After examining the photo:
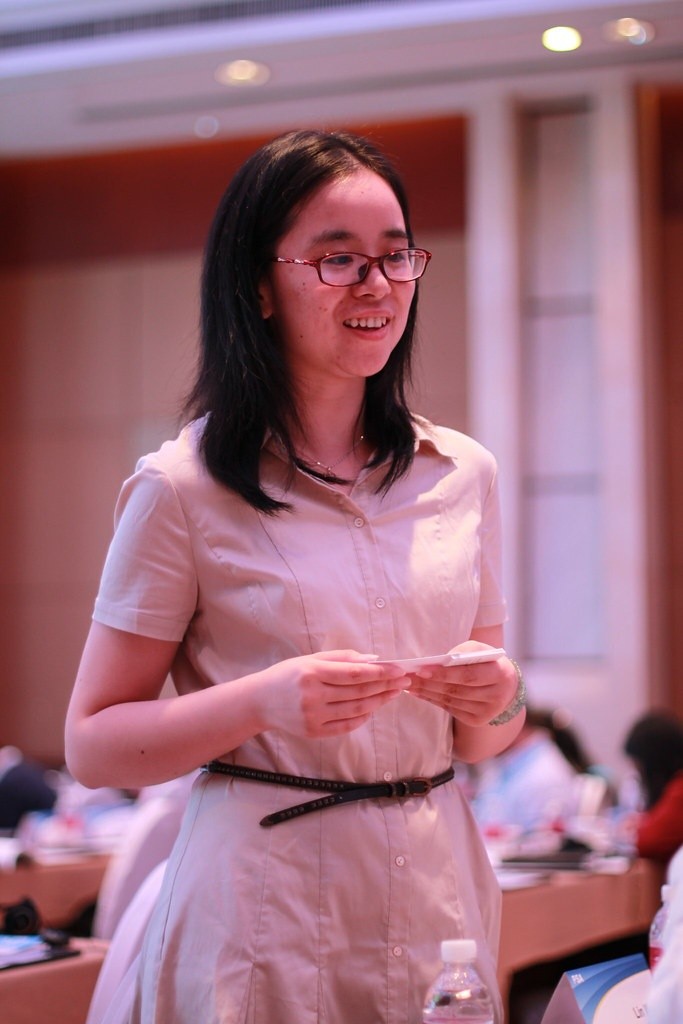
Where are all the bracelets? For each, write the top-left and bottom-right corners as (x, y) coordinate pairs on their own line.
(491, 659), (525, 725)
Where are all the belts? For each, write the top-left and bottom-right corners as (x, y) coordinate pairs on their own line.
(200, 756), (455, 828)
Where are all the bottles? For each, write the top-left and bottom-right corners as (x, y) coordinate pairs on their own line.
(421, 940), (494, 1024)
(649, 886), (671, 973)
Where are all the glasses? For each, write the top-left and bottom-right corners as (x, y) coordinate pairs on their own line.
(273, 249), (432, 286)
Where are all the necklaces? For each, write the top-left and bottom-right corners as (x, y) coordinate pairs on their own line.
(290, 440), (358, 482)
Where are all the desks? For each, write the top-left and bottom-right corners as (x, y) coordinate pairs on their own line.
(0, 847), (661, 1024)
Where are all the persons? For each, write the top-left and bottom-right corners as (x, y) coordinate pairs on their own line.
(64, 137), (526, 1024)
(0, 702), (683, 1024)
